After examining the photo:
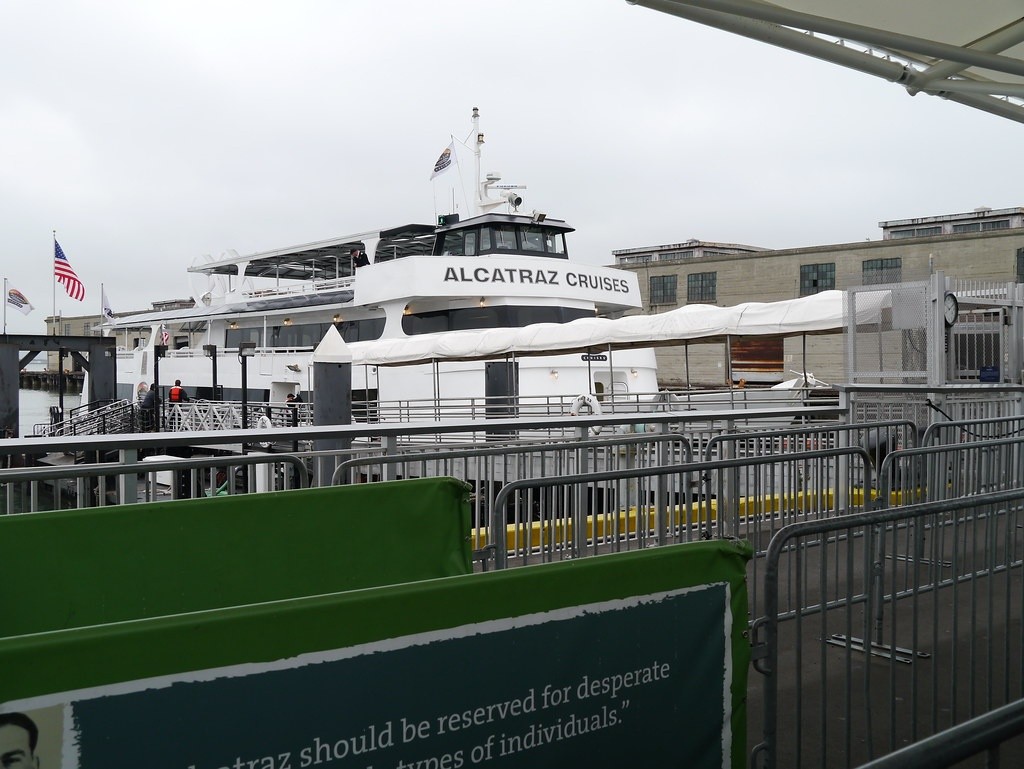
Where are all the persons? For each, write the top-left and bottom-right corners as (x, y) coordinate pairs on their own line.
(286, 393), (305, 422)
(140, 383), (162, 433)
(169, 380), (190, 432)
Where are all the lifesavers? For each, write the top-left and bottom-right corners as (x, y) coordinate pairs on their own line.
(569, 393), (603, 436)
(257, 415), (272, 449)
(64, 369), (68, 375)
(650, 390), (683, 432)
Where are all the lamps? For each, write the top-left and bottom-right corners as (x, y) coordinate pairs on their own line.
(508, 192), (522, 213)
(283, 318), (290, 326)
(479, 296), (485, 307)
(287, 365), (301, 372)
(403, 305), (408, 314)
(333, 314), (340, 322)
(230, 322), (236, 329)
(533, 209), (547, 223)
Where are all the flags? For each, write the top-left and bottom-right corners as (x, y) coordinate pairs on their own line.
(53, 234), (85, 301)
(101, 285), (116, 326)
(159, 323), (169, 345)
(4, 279), (35, 316)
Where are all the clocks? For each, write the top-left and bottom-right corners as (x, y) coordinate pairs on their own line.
(944, 290), (959, 329)
